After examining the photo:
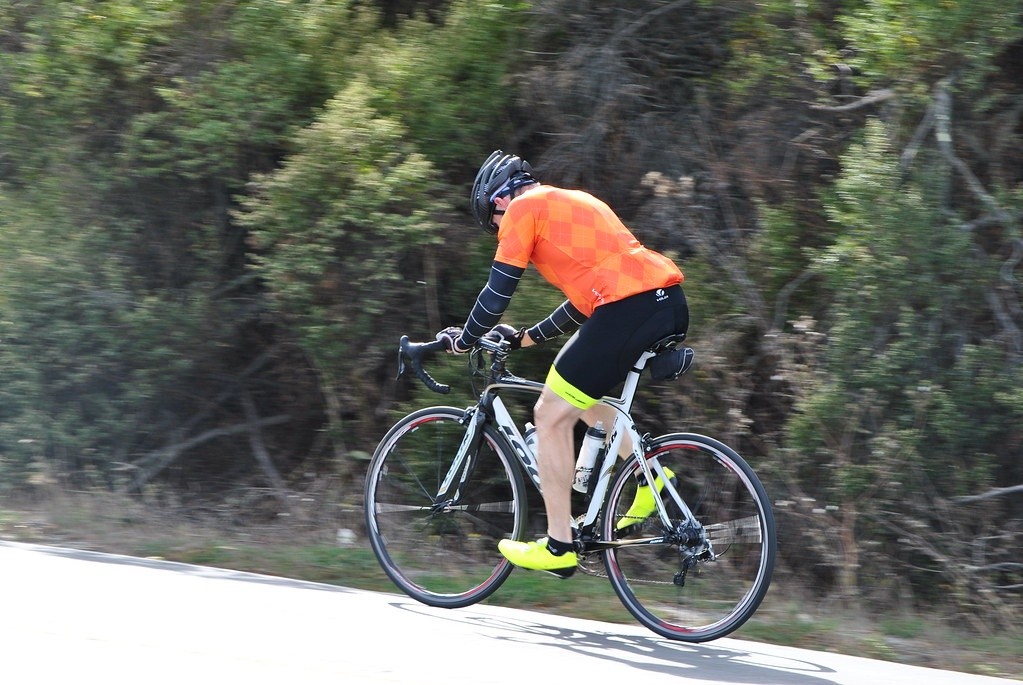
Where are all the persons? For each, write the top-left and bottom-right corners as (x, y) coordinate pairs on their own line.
(436, 150), (690, 581)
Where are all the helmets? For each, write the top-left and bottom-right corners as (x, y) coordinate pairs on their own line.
(470, 150), (535, 230)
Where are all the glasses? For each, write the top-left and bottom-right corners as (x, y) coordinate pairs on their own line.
(485, 224), (498, 235)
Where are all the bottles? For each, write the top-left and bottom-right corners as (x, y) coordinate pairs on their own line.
(571, 421), (608, 493)
(523, 422), (538, 465)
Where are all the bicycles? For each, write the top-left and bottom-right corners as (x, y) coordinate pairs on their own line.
(364, 330), (776, 644)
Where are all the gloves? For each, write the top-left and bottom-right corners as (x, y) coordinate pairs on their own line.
(436, 327), (469, 355)
(485, 323), (529, 355)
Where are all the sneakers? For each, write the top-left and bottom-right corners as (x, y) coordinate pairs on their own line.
(497, 536), (578, 577)
(616, 467), (679, 531)
